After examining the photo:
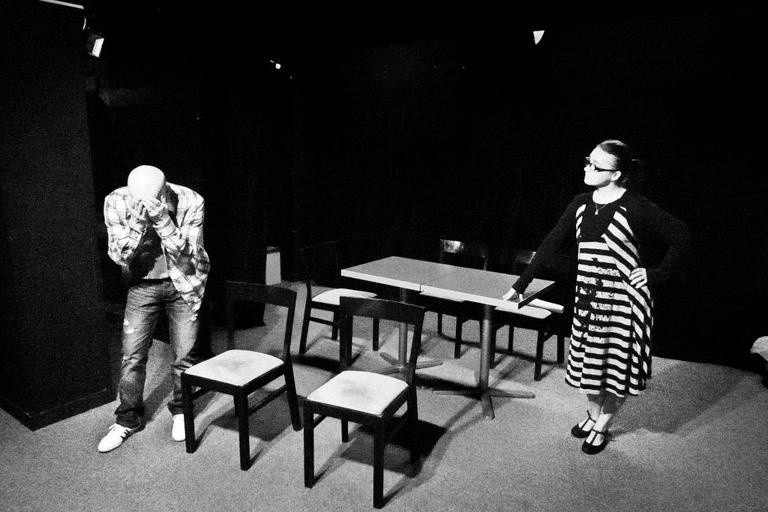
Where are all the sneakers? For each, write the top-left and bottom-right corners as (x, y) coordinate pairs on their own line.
(99, 416), (146, 455)
(171, 412), (188, 441)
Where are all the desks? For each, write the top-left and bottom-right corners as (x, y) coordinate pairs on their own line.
(340, 256), (556, 420)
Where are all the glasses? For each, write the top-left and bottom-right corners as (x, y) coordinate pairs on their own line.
(584, 156), (618, 174)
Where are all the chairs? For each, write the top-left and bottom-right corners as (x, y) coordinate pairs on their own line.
(414, 237), (496, 354)
(303, 239), (380, 366)
(303, 294), (426, 510)
(179, 281), (302, 474)
(488, 248), (569, 381)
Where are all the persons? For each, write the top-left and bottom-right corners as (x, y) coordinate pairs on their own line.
(96, 164), (210, 453)
(501, 139), (700, 454)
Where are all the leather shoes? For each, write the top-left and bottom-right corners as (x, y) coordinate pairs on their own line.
(582, 424), (609, 455)
(571, 411), (597, 437)
